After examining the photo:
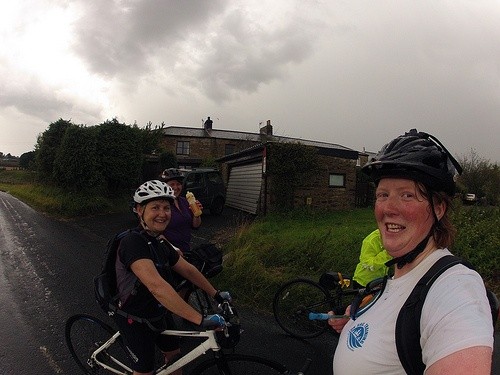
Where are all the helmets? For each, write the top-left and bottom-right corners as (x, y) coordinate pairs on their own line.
(162, 168), (185, 182)
(133, 180), (177, 205)
(361, 128), (462, 185)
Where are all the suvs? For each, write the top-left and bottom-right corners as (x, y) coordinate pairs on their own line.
(178, 168), (227, 216)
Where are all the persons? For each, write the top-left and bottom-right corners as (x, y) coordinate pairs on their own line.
(115, 180), (232, 375)
(158, 167), (203, 253)
(328, 129), (494, 375)
(352, 228), (394, 289)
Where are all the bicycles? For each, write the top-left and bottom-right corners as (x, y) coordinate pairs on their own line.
(64, 298), (289, 375)
(172, 251), (219, 327)
(273, 269), (364, 339)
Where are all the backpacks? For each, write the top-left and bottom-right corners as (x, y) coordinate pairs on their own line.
(92, 229), (160, 322)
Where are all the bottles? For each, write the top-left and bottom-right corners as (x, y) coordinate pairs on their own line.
(186, 191), (202, 217)
